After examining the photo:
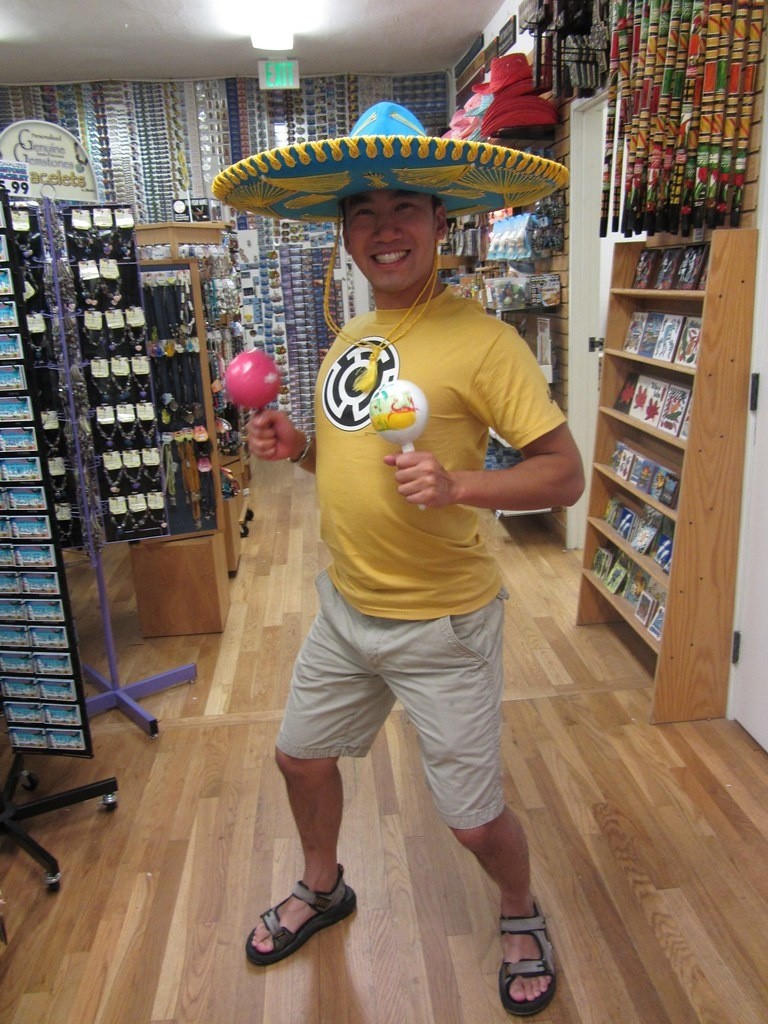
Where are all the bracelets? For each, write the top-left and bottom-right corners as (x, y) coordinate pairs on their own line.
(287, 431), (314, 466)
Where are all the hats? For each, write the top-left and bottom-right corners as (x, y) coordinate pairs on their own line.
(439, 53), (558, 143)
(210, 100), (568, 392)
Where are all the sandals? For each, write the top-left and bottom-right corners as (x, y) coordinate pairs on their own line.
(246, 863), (356, 967)
(498, 900), (557, 1017)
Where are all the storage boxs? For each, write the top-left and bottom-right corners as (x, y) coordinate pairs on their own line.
(485, 274), (561, 311)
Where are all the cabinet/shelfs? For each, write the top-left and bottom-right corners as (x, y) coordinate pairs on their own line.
(575, 230), (760, 725)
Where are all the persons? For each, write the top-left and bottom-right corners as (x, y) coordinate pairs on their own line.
(248, 102), (587, 1017)
